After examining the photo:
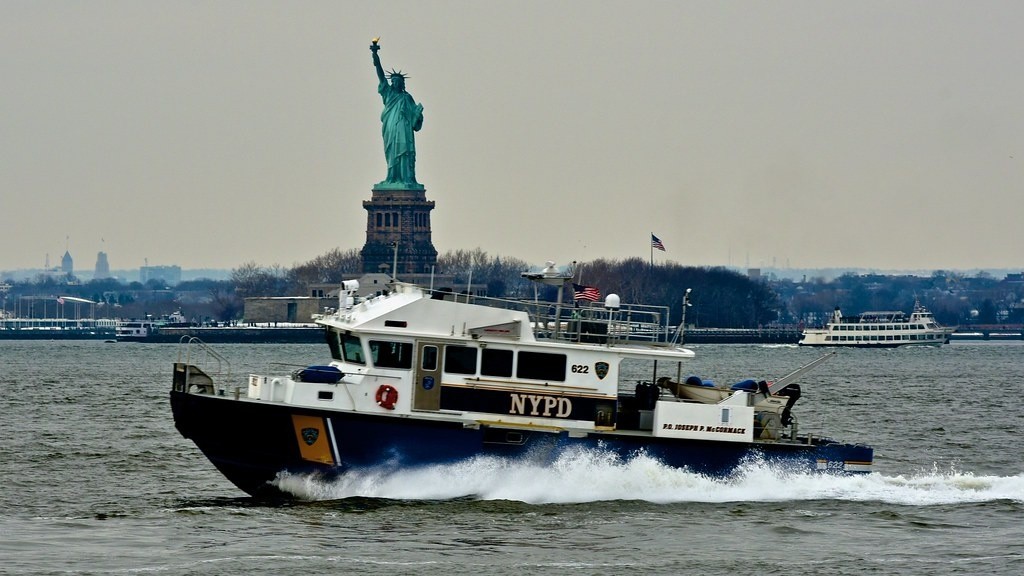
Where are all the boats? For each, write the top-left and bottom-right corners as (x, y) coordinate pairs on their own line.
(796, 287), (960, 349)
(114, 309), (187, 343)
(166, 238), (874, 504)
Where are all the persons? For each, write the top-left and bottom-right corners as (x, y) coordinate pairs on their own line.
(372, 51), (424, 187)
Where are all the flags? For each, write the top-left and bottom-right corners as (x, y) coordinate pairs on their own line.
(56, 295), (64, 305)
(571, 283), (600, 302)
(650, 233), (666, 252)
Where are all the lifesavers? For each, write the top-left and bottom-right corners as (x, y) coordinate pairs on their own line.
(376, 385), (398, 407)
